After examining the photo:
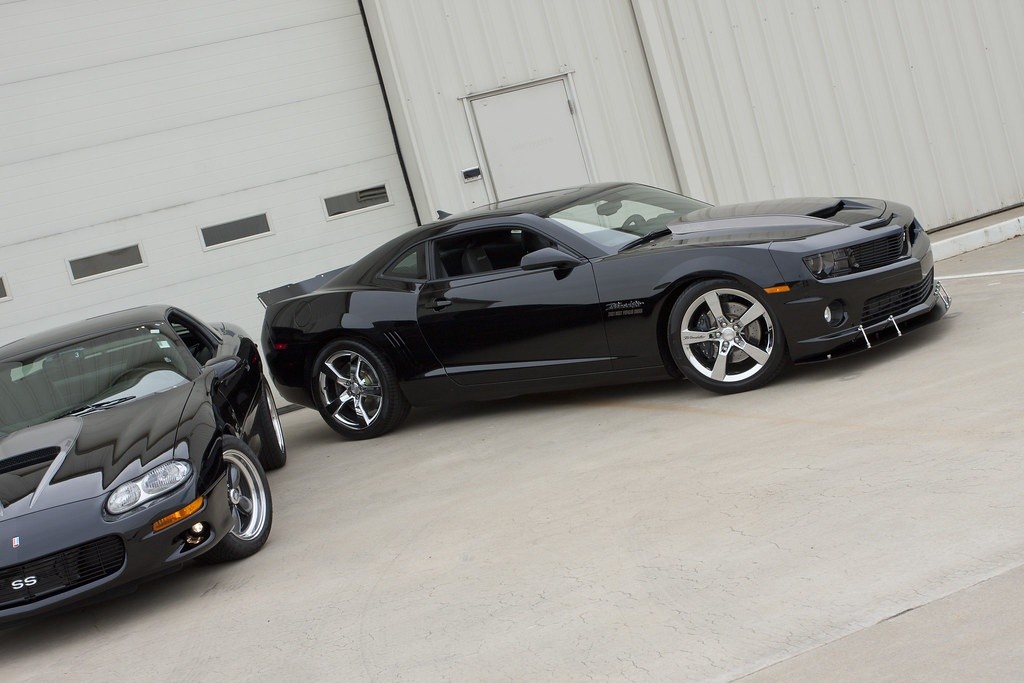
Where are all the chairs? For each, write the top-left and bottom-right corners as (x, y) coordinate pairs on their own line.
(521, 231), (557, 254)
(41, 351), (87, 380)
(462, 245), (493, 276)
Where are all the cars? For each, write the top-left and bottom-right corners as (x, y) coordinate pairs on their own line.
(0, 305), (286, 627)
(258, 180), (949, 441)
